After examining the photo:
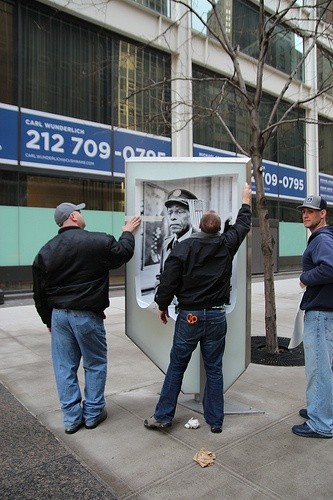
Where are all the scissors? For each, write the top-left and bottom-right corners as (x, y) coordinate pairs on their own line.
(186, 314), (198, 324)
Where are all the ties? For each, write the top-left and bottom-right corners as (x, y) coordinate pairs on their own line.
(172, 240), (177, 249)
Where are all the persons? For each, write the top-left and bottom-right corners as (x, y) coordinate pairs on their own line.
(158, 189), (198, 275)
(32, 203), (141, 435)
(144, 182), (252, 433)
(292, 194), (333, 439)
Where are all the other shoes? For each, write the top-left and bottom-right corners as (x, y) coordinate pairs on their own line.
(65, 422), (84, 434)
(86, 410), (107, 429)
(144, 416), (172, 428)
(211, 426), (222, 433)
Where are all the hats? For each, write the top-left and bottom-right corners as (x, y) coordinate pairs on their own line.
(165, 188), (198, 210)
(296, 196), (327, 210)
(54, 202), (85, 226)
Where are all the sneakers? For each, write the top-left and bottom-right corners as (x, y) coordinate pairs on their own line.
(299, 409), (310, 419)
(292, 422), (332, 438)
